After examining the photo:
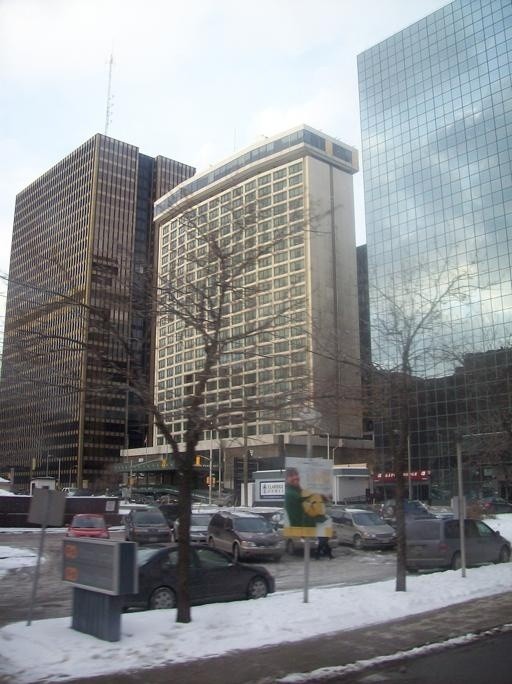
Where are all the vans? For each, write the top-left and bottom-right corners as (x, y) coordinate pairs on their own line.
(63, 488), (79, 496)
(331, 509), (396, 550)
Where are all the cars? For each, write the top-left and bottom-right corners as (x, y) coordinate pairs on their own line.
(405, 500), (512, 572)
(65, 514), (111, 538)
(125, 502), (339, 561)
(121, 543), (275, 613)
(479, 497), (512, 513)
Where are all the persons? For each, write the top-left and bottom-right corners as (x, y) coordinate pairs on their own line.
(315, 537), (335, 561)
(285, 465), (329, 527)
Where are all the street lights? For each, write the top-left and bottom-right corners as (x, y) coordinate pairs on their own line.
(46, 455), (78, 487)
(192, 455), (213, 504)
